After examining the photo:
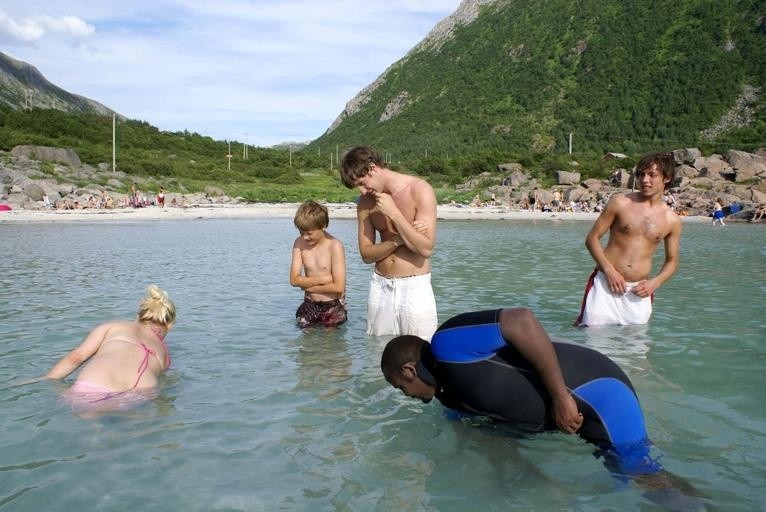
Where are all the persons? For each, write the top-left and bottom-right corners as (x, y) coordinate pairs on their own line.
(449, 185), (611, 214)
(585, 323), (672, 392)
(750, 205), (766, 221)
(710, 199), (726, 227)
(291, 329), (358, 499)
(666, 190), (675, 211)
(378, 302), (713, 512)
(15, 282), (176, 420)
(51, 180), (165, 211)
(569, 151), (683, 329)
(288, 199), (347, 327)
(340, 143), (438, 344)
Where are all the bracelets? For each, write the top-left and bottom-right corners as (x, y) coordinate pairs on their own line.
(390, 236), (399, 249)
